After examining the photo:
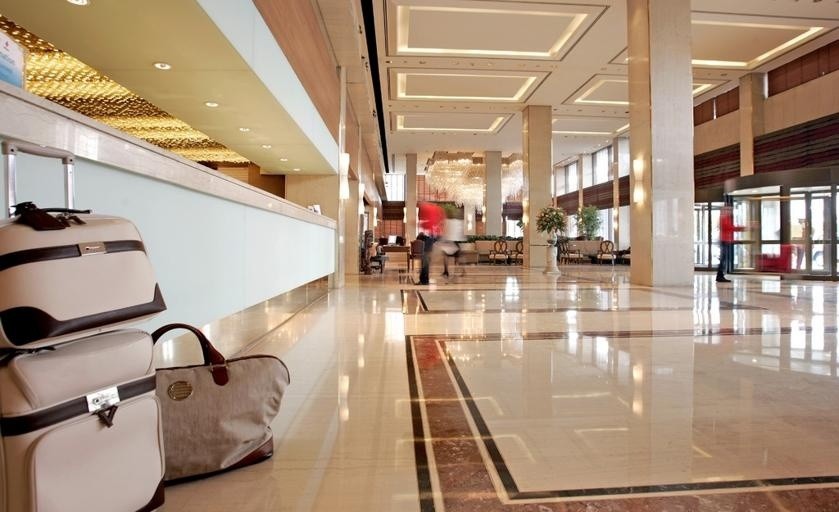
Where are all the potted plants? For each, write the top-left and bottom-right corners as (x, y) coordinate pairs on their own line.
(535, 205), (569, 245)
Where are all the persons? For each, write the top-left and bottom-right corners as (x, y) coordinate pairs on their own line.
(792, 219), (814, 269)
(440, 207), (467, 278)
(415, 198), (446, 286)
(714, 204), (758, 283)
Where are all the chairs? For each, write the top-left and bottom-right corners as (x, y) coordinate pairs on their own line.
(489, 238), (524, 266)
(557, 238), (630, 265)
(408, 239), (425, 271)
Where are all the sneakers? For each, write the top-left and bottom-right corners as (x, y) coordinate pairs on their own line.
(414, 281), (429, 285)
(716, 276), (731, 282)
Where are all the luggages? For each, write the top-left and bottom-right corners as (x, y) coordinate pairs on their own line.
(1, 138), (166, 511)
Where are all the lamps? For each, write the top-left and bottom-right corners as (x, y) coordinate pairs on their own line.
(420, 151), (523, 215)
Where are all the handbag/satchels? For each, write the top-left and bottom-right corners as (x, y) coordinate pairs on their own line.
(0, 200), (167, 352)
(151, 322), (291, 486)
